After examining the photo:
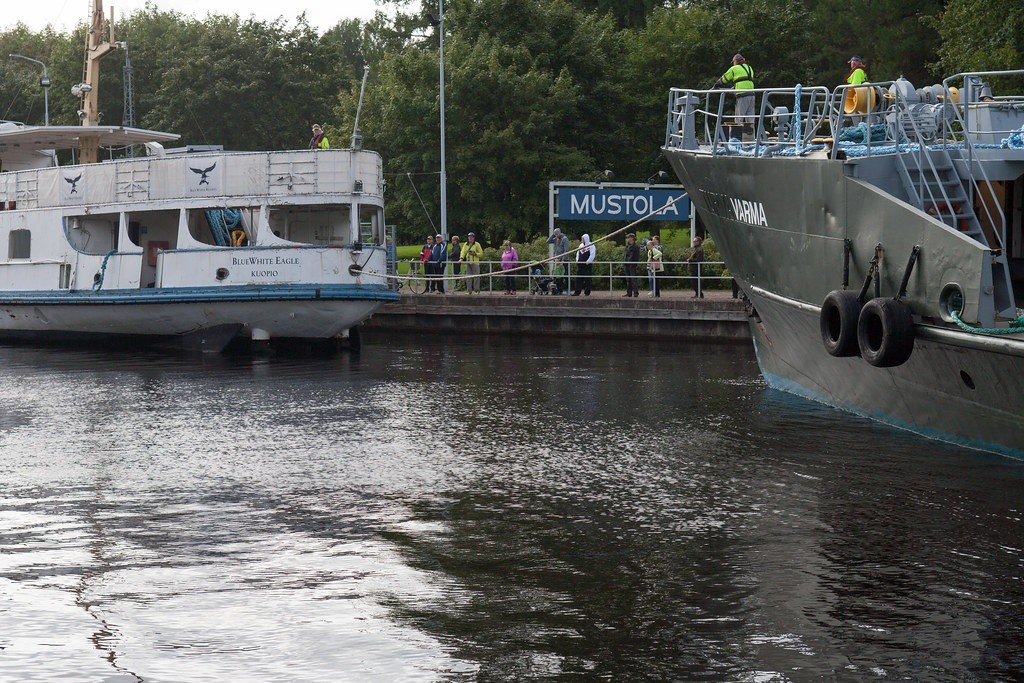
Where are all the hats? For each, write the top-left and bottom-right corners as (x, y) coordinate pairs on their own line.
(427, 236), (433, 240)
(435, 235), (442, 238)
(554, 228), (561, 235)
(731, 54), (745, 65)
(848, 56), (862, 63)
(451, 236), (460, 241)
(467, 233), (475, 237)
(626, 233), (635, 238)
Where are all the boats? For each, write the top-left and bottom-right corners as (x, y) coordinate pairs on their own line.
(662, 70), (1024, 464)
(1, 0), (404, 352)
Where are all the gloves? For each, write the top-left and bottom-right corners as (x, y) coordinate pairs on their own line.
(458, 258), (463, 264)
(469, 251), (476, 256)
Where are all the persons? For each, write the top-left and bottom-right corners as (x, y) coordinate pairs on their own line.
(458, 233), (484, 294)
(845, 55), (867, 126)
(309, 124), (329, 149)
(711, 53), (757, 145)
(646, 236), (664, 298)
(501, 241), (518, 295)
(367, 234), (448, 294)
(686, 236), (706, 299)
(622, 233), (640, 297)
(570, 233), (596, 296)
(448, 236), (462, 288)
(535, 228), (570, 295)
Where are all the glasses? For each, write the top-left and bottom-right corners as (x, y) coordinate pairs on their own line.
(692, 239), (697, 243)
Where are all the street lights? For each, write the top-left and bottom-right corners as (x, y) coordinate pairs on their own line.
(7, 53), (49, 126)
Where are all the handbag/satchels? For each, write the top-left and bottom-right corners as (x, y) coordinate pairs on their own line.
(651, 250), (660, 270)
(553, 262), (565, 278)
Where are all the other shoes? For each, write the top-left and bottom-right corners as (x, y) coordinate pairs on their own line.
(691, 292), (704, 299)
(437, 292), (445, 294)
(423, 289), (429, 293)
(473, 291), (479, 295)
(622, 293), (631, 297)
(571, 293), (579, 296)
(647, 291), (653, 297)
(429, 289), (434, 293)
(634, 292), (638, 297)
(511, 290), (516, 295)
(504, 290), (510, 295)
(585, 294), (589, 296)
(465, 291), (472, 296)
